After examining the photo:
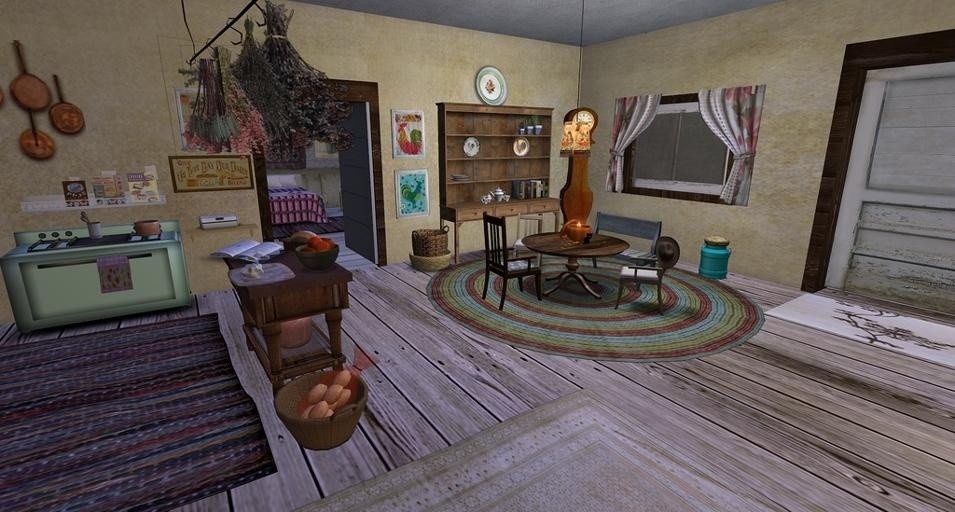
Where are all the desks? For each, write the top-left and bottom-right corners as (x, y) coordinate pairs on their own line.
(222, 240), (354, 400)
(437, 196), (560, 267)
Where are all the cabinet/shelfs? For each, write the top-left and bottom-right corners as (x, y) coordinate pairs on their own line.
(435, 101), (555, 202)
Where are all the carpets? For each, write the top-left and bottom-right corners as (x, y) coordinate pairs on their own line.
(421, 253), (770, 365)
(1, 311), (285, 510)
(763, 289), (954, 371)
(286, 384), (777, 511)
(267, 211), (345, 238)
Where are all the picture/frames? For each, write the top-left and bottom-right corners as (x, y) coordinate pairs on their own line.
(390, 108), (425, 160)
(167, 155), (255, 193)
(394, 168), (430, 220)
(173, 87), (206, 152)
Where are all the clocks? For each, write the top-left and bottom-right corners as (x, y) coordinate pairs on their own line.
(564, 106), (600, 146)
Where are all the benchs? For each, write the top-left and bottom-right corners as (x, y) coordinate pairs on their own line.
(592, 209), (662, 289)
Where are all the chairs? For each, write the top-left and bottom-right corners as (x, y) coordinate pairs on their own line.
(481, 210), (546, 312)
(509, 212), (546, 274)
(614, 253), (675, 321)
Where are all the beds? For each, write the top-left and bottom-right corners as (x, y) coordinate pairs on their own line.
(264, 183), (331, 225)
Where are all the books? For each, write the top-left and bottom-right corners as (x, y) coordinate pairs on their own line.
(209, 239), (283, 262)
